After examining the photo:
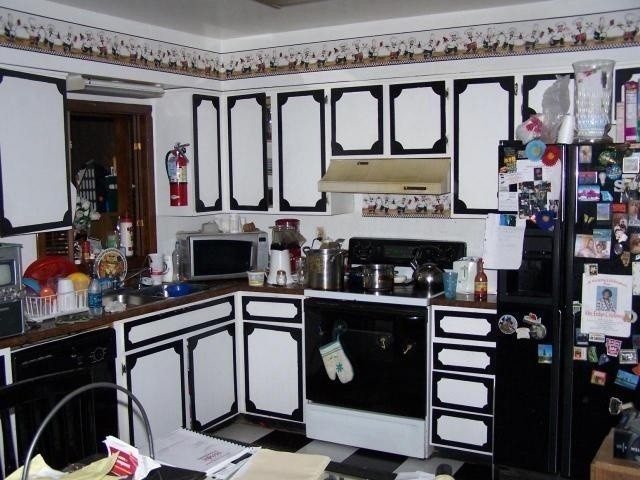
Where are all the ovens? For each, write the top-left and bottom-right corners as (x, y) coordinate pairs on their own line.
(302, 292), (435, 420)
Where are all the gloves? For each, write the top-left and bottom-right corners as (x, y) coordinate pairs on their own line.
(319, 341), (354, 383)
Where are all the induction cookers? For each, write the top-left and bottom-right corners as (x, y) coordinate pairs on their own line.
(304, 272), (445, 299)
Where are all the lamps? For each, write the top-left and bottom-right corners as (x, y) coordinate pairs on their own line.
(65, 71), (165, 99)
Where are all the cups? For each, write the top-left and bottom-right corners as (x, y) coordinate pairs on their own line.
(442, 271), (458, 299)
(147, 253), (164, 286)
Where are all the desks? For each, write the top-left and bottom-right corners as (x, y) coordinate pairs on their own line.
(105, 427), (397, 480)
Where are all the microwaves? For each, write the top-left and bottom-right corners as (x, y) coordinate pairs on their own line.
(175, 230), (269, 281)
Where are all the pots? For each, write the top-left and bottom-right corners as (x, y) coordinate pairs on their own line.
(353, 262), (399, 291)
(302, 245), (345, 291)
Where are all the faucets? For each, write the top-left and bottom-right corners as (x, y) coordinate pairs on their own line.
(113, 266), (152, 292)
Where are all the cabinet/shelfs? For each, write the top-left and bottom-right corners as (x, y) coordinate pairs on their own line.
(115, 292), (240, 455)
(429, 304), (498, 462)
(238, 291), (304, 431)
(590, 425), (640, 480)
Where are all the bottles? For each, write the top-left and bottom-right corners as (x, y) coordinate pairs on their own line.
(295, 256), (305, 285)
(87, 274), (103, 319)
(474, 258), (488, 303)
(119, 216), (134, 257)
(171, 241), (186, 282)
(276, 270), (287, 286)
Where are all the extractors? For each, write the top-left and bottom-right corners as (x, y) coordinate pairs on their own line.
(317, 157), (451, 196)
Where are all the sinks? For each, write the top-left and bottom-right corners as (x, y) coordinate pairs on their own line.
(132, 283), (225, 300)
(101, 288), (160, 315)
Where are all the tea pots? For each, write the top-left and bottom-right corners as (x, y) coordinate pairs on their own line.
(409, 245), (444, 290)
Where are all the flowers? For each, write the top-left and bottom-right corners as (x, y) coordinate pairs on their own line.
(72, 197), (101, 240)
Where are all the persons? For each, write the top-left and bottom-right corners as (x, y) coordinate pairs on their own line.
(596, 289), (615, 312)
(578, 239), (594, 257)
(595, 244), (606, 258)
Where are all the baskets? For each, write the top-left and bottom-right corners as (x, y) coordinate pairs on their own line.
(23, 289), (88, 323)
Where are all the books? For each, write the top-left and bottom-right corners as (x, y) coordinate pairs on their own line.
(140, 426), (253, 475)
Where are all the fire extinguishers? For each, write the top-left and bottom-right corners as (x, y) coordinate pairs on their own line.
(165, 140), (191, 207)
